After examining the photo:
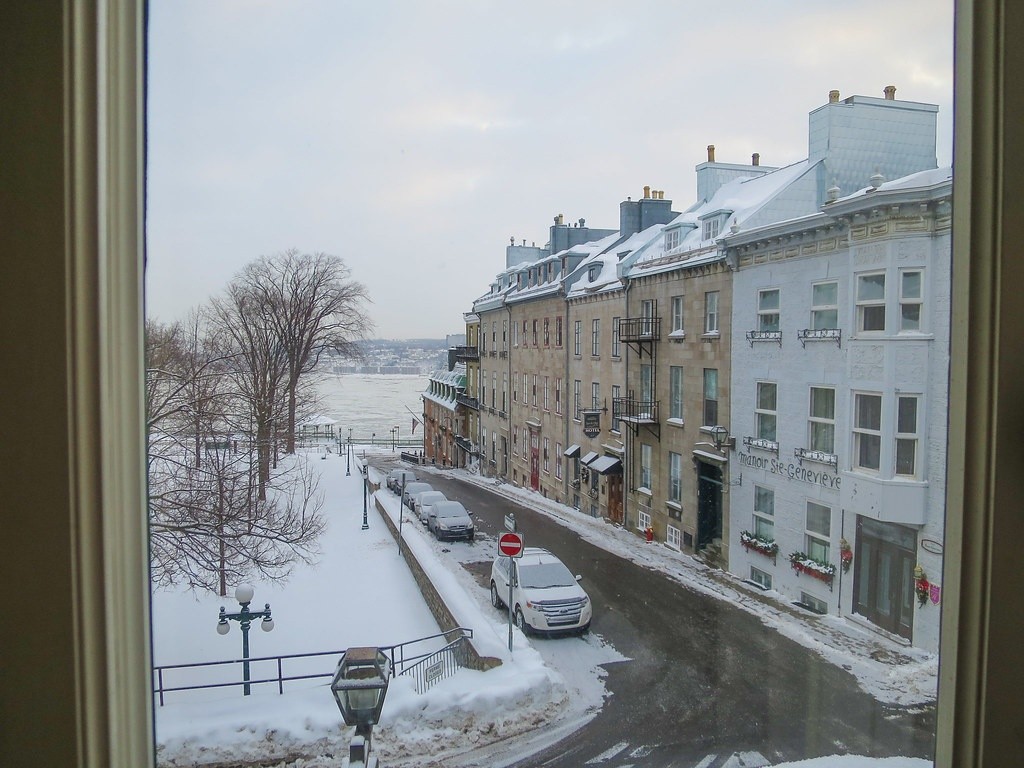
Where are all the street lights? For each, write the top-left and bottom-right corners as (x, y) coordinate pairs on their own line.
(348, 427), (353, 443)
(361, 459), (369, 530)
(337, 424), (343, 456)
(216, 584), (275, 696)
(390, 427), (396, 452)
(345, 433), (353, 476)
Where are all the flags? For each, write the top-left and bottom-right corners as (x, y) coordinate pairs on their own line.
(412, 418), (419, 434)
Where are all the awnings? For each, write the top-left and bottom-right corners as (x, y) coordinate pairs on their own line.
(587, 455), (623, 475)
(580, 451), (600, 466)
(564, 443), (580, 458)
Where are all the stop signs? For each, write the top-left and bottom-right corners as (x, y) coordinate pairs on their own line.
(499, 533), (523, 557)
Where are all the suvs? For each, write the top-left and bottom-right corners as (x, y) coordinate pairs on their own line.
(490, 547), (592, 635)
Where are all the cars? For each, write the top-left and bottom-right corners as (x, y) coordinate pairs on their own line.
(395, 472), (419, 496)
(386, 468), (410, 491)
(428, 501), (475, 542)
(403, 483), (433, 510)
(414, 492), (448, 525)
